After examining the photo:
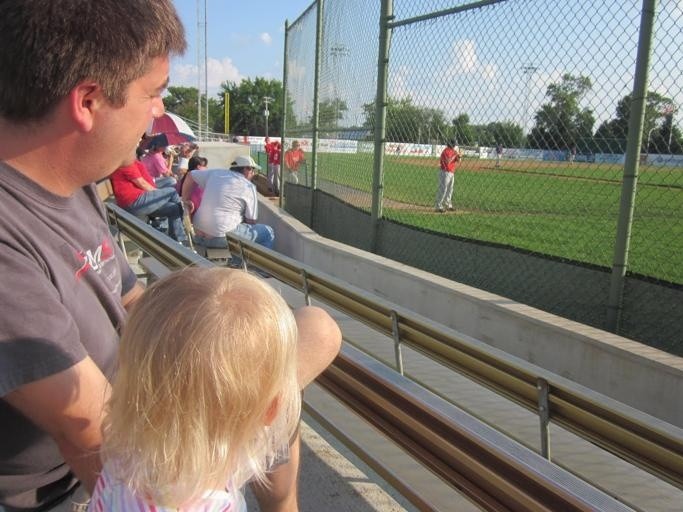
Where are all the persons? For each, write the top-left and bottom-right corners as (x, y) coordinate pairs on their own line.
(396, 145), (401, 156)
(569, 143), (576, 165)
(435, 138), (463, 213)
(495, 142), (503, 168)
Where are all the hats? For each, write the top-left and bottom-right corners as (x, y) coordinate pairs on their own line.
(447, 139), (456, 145)
(230, 155), (261, 170)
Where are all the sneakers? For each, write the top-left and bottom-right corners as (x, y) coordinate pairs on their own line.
(437, 205), (455, 213)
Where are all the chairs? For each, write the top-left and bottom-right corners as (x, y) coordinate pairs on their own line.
(97, 179), (234, 270)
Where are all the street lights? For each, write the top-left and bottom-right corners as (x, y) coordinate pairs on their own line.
(521, 64), (540, 149)
(329, 43), (351, 139)
(263, 96), (276, 137)
